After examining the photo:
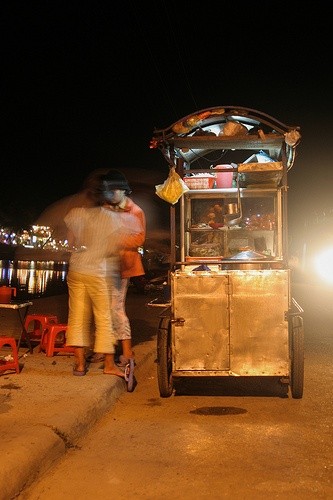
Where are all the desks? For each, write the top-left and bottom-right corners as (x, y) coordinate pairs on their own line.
(0, 301), (33, 354)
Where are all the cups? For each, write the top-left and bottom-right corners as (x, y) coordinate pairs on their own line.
(215, 164), (233, 188)
(0, 286), (16, 304)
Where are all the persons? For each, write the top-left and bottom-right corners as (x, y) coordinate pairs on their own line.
(65, 180), (142, 376)
(89, 170), (147, 364)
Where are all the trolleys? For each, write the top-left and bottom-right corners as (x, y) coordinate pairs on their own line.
(147, 105), (306, 399)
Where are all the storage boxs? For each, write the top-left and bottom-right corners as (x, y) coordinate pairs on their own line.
(184, 177), (217, 190)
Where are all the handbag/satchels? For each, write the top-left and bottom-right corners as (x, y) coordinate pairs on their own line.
(155, 166), (189, 205)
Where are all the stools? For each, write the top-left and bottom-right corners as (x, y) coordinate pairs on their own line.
(22, 313), (59, 342)
(40, 323), (76, 357)
(0, 335), (20, 375)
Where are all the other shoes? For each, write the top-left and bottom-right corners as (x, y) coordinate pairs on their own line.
(116, 356), (136, 367)
(86, 353), (105, 363)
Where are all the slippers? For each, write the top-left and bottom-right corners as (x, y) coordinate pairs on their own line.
(124, 358), (134, 393)
(73, 360), (89, 376)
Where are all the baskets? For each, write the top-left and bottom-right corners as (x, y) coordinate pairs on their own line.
(182, 177), (216, 189)
